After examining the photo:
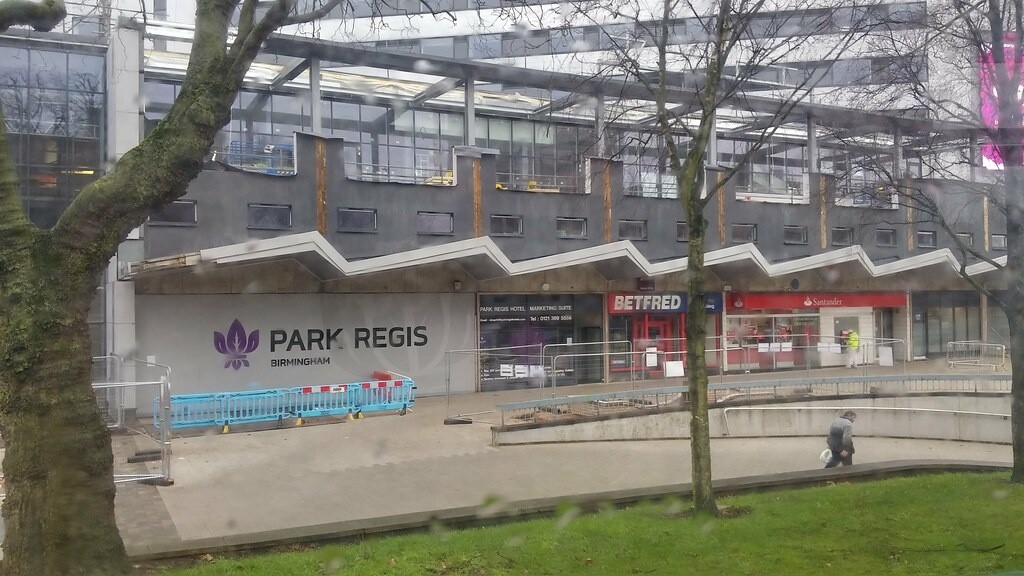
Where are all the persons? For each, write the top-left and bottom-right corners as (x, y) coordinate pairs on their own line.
(846, 328), (859, 368)
(824, 410), (858, 468)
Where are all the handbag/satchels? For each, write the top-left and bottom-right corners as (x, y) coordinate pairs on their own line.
(819, 449), (833, 463)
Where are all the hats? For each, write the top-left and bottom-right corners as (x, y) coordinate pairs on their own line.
(847, 328), (853, 334)
